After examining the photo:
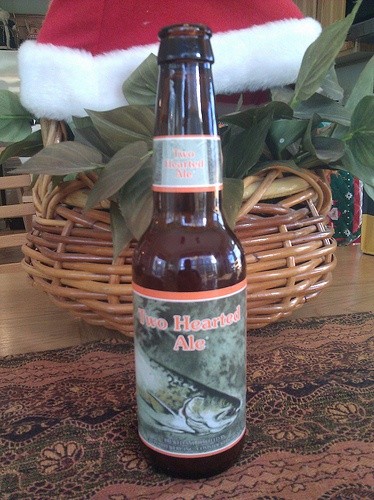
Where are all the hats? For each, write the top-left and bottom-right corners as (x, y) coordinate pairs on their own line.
(19, 0), (324, 123)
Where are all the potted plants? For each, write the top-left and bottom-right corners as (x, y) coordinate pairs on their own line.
(0, 1), (374, 335)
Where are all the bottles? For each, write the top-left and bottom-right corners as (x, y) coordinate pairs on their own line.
(125, 21), (249, 479)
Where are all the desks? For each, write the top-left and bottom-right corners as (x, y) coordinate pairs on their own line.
(1, 252), (373, 499)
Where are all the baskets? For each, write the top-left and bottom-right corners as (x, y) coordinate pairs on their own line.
(18, 113), (335, 339)
